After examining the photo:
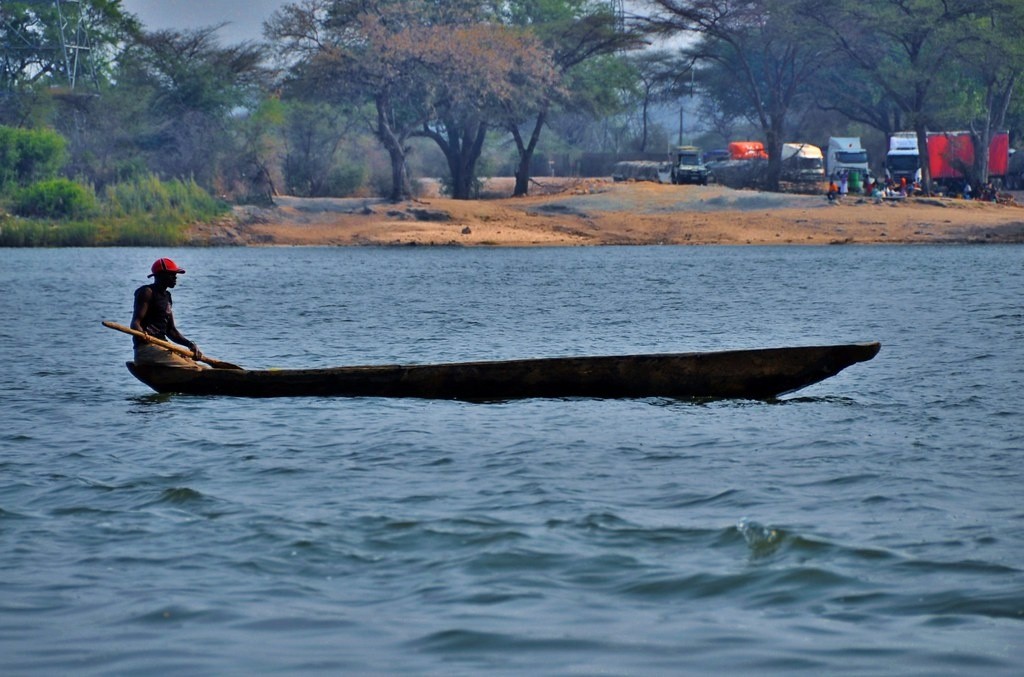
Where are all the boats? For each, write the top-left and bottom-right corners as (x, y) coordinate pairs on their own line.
(125, 342), (882, 400)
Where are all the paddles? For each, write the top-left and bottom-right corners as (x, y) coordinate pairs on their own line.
(102, 318), (242, 369)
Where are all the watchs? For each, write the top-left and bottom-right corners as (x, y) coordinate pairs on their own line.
(188, 341), (194, 349)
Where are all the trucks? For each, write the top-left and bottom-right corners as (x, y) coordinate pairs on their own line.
(886, 130), (1024, 191)
(670, 145), (708, 185)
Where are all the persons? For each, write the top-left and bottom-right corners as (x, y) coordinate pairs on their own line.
(130, 258), (203, 365)
(827, 170), (1000, 201)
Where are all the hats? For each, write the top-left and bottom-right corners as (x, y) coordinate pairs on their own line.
(147, 258), (185, 278)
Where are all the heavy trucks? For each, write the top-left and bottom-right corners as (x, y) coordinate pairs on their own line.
(612, 160), (672, 184)
(705, 138), (870, 193)
